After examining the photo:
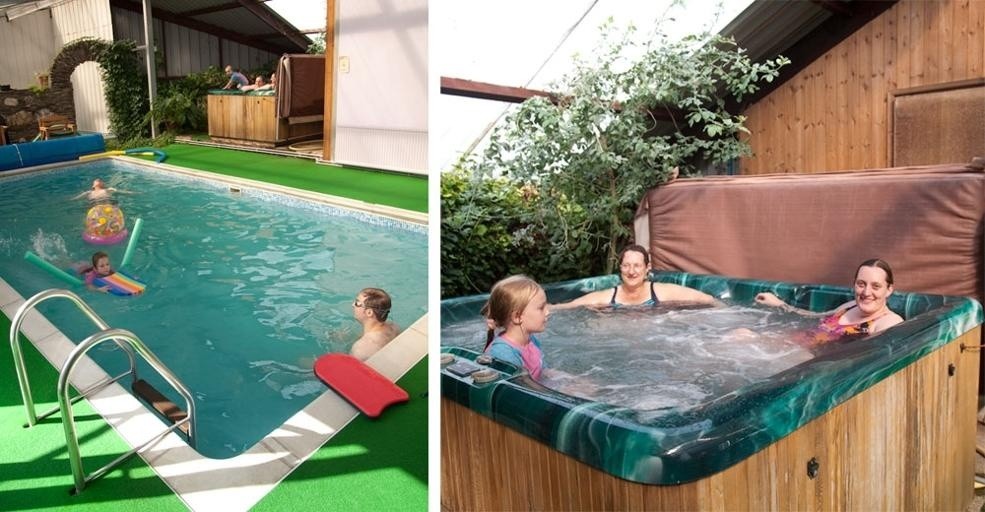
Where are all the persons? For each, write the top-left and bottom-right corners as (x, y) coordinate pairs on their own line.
(299, 287), (404, 376)
(548, 243), (732, 313)
(68, 176), (140, 208)
(56, 251), (112, 295)
(714, 256), (902, 376)
(239, 75), (265, 94)
(253, 73), (276, 91)
(477, 275), (595, 400)
(219, 63), (247, 93)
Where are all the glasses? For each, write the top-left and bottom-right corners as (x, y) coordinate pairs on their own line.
(353, 300), (371, 308)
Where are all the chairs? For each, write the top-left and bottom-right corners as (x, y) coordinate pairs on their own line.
(38, 114), (76, 141)
